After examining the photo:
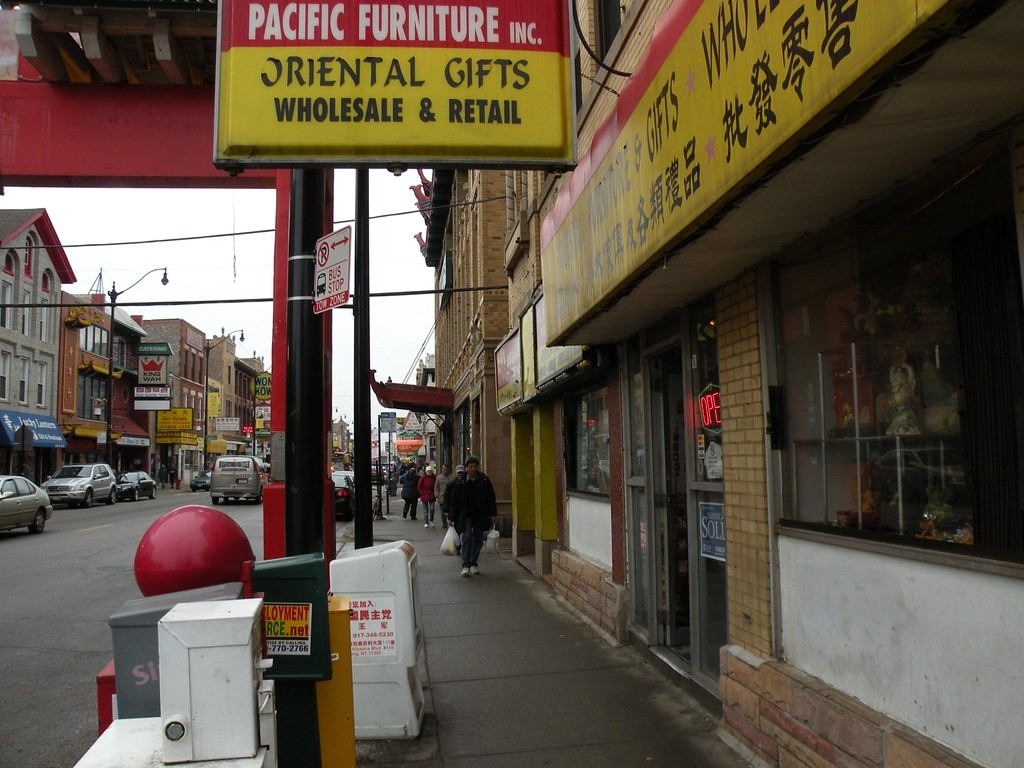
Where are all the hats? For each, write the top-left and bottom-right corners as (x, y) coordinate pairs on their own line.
(426, 466), (432, 471)
(455, 465), (464, 472)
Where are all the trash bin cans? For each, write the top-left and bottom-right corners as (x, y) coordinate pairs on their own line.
(329, 540), (428, 742)
(108, 580), (245, 720)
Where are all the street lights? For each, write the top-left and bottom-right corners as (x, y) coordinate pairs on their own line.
(104, 266), (170, 466)
(202, 327), (247, 470)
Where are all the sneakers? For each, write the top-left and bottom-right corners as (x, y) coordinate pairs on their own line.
(461, 568), (470, 577)
(469, 566), (479, 574)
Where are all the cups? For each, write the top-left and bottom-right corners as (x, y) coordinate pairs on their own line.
(837, 511), (851, 526)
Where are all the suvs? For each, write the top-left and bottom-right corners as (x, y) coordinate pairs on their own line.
(38, 463), (118, 508)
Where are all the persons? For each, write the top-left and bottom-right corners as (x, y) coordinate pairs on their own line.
(22, 462), (31, 476)
(399, 464), (453, 528)
(159, 464), (168, 489)
(448, 458), (497, 576)
(169, 468), (176, 489)
(444, 464), (466, 556)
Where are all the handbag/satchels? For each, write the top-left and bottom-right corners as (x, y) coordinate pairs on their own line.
(487, 524), (499, 548)
(440, 522), (462, 558)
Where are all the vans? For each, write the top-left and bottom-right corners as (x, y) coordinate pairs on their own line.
(210, 455), (269, 505)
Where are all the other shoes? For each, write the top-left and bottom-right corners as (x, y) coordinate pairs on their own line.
(403, 514), (406, 518)
(442, 524), (447, 528)
(424, 524), (429, 527)
(411, 516), (417, 519)
(430, 521), (434, 527)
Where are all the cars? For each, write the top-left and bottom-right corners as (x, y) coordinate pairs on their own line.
(333, 465), (388, 522)
(190, 471), (214, 493)
(97, 471), (157, 501)
(0, 475), (53, 534)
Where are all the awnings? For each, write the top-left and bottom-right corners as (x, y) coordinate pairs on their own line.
(0, 409), (68, 448)
